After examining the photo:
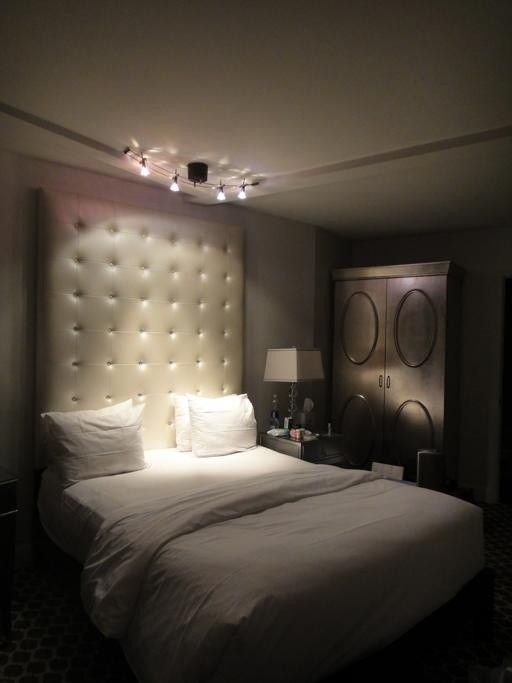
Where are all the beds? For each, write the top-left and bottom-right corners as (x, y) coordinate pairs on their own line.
(31, 438), (487, 682)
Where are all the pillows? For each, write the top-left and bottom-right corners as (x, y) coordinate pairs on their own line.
(37, 396), (149, 483)
(173, 391), (258, 457)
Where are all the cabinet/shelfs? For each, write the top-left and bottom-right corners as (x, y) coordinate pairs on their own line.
(327, 260), (469, 492)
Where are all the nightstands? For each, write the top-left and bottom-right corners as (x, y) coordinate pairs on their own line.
(1, 465), (22, 636)
(259, 429), (346, 469)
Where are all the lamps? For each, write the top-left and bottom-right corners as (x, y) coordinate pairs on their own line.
(262, 347), (326, 435)
(123, 145), (260, 201)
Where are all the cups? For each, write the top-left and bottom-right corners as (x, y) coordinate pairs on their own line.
(294, 428), (305, 442)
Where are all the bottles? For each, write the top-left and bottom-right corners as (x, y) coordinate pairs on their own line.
(267, 395), (280, 432)
(327, 423), (335, 437)
